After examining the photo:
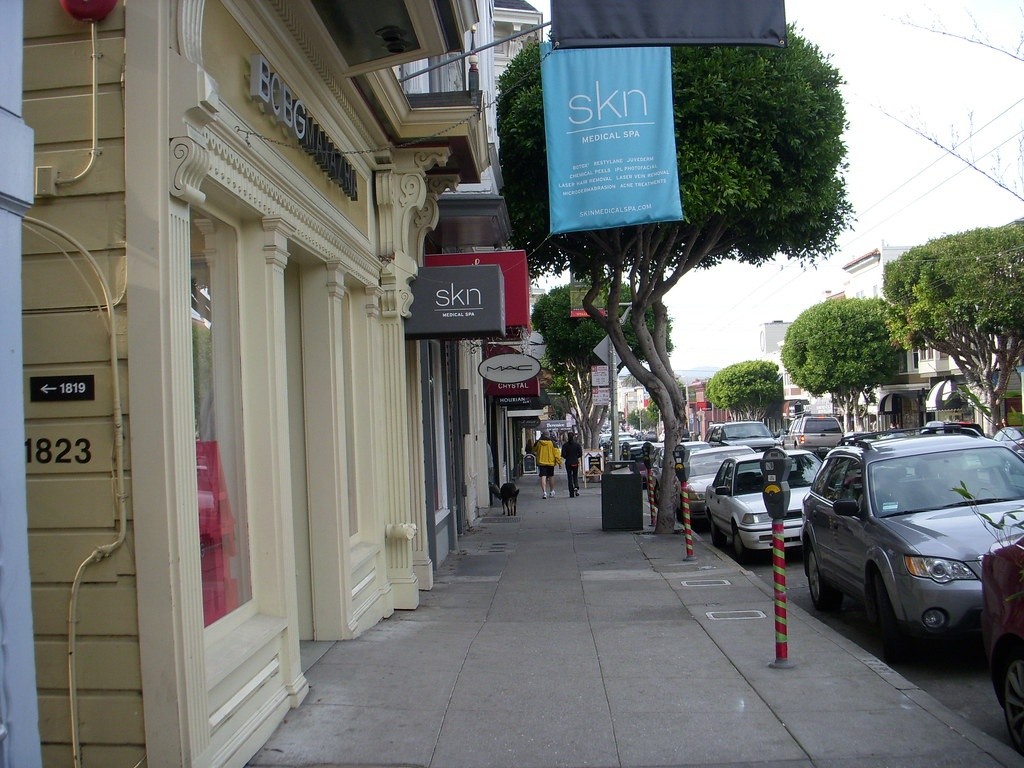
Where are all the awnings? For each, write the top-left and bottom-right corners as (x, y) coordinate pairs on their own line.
(924, 380), (966, 411)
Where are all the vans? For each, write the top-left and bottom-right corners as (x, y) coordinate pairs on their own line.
(780, 413), (846, 460)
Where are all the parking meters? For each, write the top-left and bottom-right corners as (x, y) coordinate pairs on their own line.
(601, 442), (610, 468)
(669, 443), (700, 560)
(642, 441), (658, 527)
(759, 445), (797, 668)
(622, 442), (631, 467)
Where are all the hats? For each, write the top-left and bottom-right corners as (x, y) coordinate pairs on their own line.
(543, 431), (549, 437)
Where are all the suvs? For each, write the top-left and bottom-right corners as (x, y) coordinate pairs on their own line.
(797, 424), (1024, 659)
(711, 419), (782, 452)
(921, 418), (988, 440)
(701, 420), (725, 446)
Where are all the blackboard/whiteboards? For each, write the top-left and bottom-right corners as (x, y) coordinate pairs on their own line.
(523, 454), (537, 473)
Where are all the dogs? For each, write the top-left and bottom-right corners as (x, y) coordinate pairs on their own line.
(500, 481), (520, 516)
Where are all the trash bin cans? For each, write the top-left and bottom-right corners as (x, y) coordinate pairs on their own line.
(588, 456), (601, 471)
(600, 460), (644, 533)
(691, 432), (696, 441)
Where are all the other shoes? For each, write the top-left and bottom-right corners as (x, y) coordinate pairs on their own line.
(549, 492), (554, 497)
(543, 494), (547, 499)
(574, 488), (580, 496)
(570, 494), (574, 497)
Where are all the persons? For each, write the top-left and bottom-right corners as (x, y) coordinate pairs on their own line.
(532, 431), (562, 499)
(560, 432), (583, 499)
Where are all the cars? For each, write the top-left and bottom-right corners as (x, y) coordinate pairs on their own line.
(598, 429), (712, 509)
(979, 527), (1024, 758)
(993, 425), (1024, 459)
(673, 444), (759, 533)
(701, 447), (828, 564)
